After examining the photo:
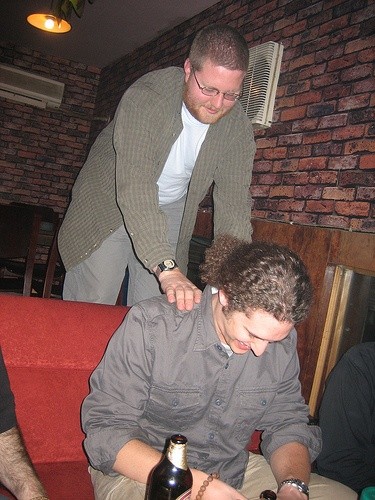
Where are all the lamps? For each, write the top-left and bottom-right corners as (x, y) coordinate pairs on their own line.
(27, 0), (83, 35)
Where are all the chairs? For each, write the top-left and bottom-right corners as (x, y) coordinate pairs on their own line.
(0, 201), (64, 301)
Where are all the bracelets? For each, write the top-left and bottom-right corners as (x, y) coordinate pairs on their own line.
(196, 472), (220, 500)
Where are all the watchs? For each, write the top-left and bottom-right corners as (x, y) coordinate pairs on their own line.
(277, 478), (309, 500)
(155, 259), (178, 278)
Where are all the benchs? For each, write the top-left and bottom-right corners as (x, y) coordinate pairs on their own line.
(0, 292), (265, 499)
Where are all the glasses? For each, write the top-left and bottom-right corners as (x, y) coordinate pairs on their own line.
(189, 64), (244, 102)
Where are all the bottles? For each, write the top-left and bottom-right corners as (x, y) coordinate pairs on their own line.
(146, 434), (193, 500)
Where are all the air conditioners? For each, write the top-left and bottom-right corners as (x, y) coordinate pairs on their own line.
(0, 63), (66, 109)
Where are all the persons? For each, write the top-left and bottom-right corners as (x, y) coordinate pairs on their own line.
(81, 234), (357, 500)
(57, 24), (256, 306)
(0, 350), (52, 500)
(314, 341), (375, 500)
